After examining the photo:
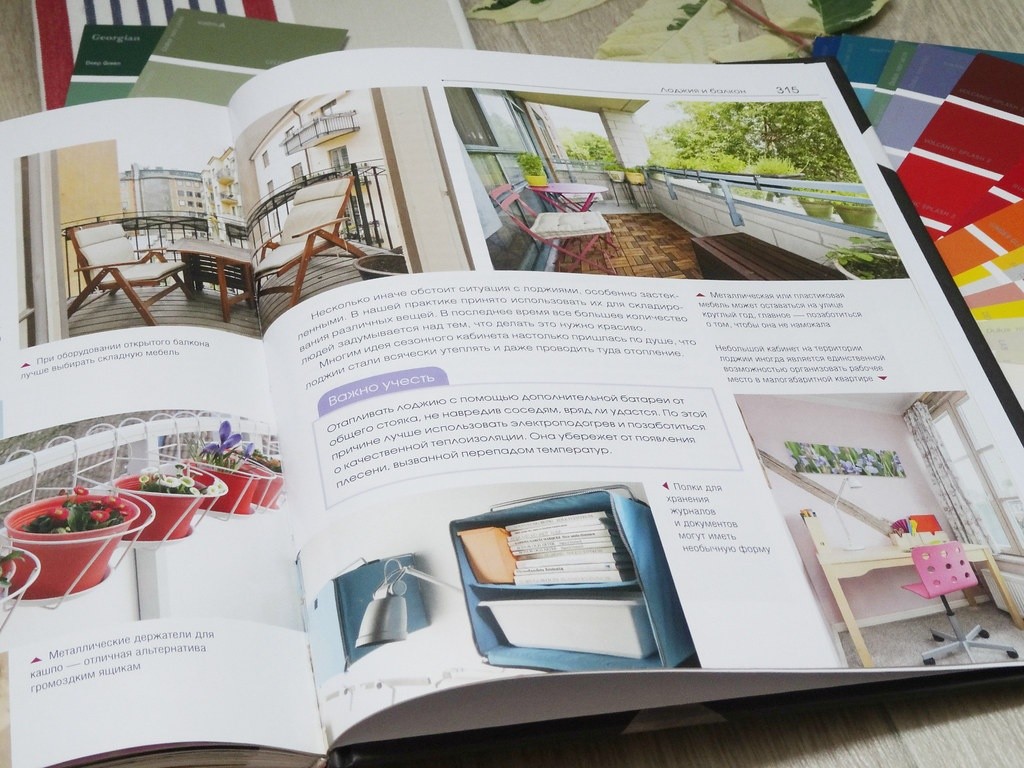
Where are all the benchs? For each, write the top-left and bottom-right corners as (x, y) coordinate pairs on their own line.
(690, 233), (849, 280)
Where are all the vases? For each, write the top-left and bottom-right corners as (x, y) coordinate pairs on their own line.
(354, 253), (411, 281)
(0, 421), (284, 610)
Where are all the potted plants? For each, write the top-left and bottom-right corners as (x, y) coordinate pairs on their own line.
(667, 149), (877, 230)
(513, 150), (550, 187)
(603, 162), (649, 186)
(819, 234), (911, 280)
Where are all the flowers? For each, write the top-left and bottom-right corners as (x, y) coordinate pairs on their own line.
(21, 478), (132, 534)
(174, 411), (262, 476)
(139, 459), (227, 497)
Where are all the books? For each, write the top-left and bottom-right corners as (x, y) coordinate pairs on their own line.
(501, 512), (629, 584)
(0, 47), (1024, 768)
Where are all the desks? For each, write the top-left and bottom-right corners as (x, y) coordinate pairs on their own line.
(164, 237), (258, 324)
(530, 183), (626, 253)
(798, 507), (1023, 670)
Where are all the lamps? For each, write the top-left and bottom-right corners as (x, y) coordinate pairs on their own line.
(354, 556), (467, 650)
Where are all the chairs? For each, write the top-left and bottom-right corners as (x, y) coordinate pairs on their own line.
(902, 541), (1019, 665)
(67, 220), (193, 327)
(488, 183), (622, 276)
(250, 176), (369, 309)
